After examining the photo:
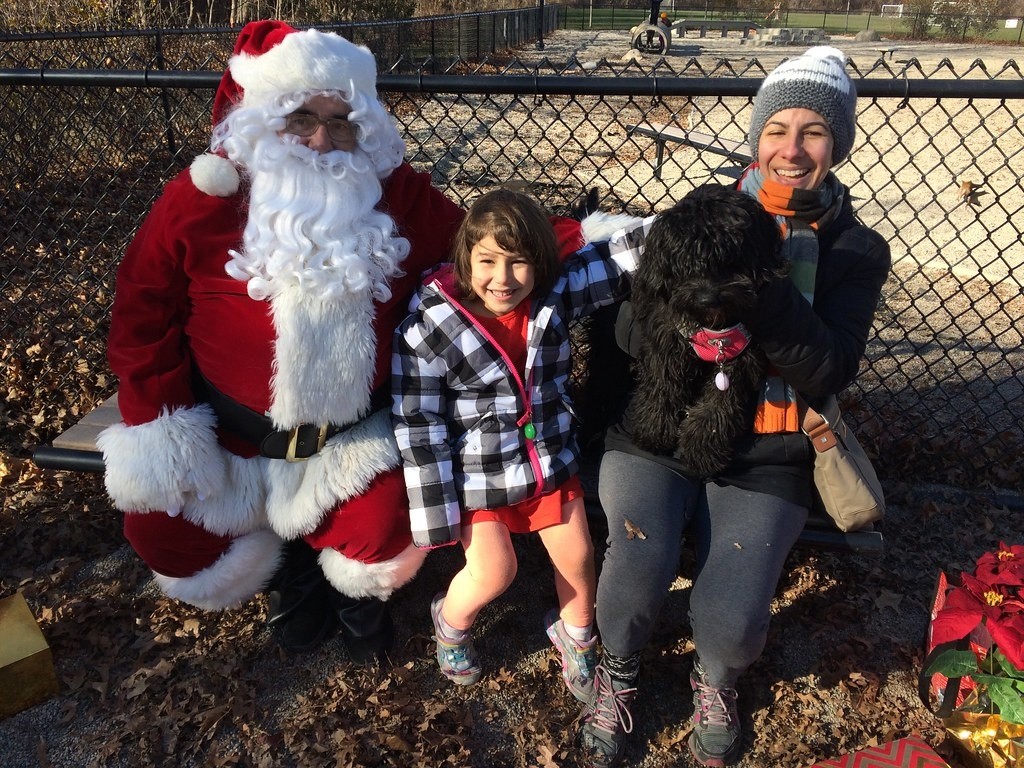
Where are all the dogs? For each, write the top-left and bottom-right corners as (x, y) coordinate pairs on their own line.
(622, 184), (787, 486)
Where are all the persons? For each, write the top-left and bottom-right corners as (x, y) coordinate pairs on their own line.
(98, 19), (649, 658)
(390, 192), (660, 702)
(584, 46), (894, 766)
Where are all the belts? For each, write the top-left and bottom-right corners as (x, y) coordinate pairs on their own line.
(188, 351), (394, 464)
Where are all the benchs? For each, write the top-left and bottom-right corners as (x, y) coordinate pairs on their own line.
(34, 392), (885, 594)
(624, 122), (754, 182)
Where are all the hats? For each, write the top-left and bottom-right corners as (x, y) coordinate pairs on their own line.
(748, 46), (858, 168)
(188, 15), (378, 195)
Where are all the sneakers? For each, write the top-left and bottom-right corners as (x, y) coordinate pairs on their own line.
(543, 608), (598, 704)
(579, 656), (639, 767)
(689, 665), (743, 767)
(430, 590), (482, 685)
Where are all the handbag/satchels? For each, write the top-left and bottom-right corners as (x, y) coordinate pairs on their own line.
(791, 388), (887, 533)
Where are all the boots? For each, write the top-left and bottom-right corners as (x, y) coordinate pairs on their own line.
(264, 542), (340, 651)
(328, 583), (399, 668)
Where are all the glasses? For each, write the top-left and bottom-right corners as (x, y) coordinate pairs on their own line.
(283, 111), (359, 143)
